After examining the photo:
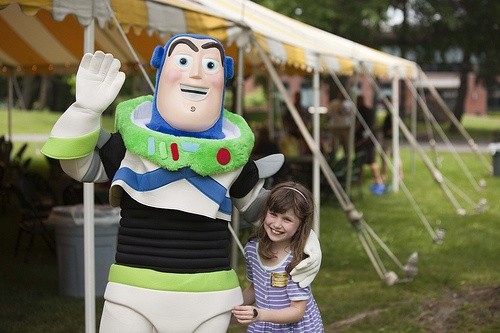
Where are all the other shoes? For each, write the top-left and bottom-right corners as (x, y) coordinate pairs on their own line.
(372, 183), (386, 195)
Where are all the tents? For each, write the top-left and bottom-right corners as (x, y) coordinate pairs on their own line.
(0, 0), (419, 333)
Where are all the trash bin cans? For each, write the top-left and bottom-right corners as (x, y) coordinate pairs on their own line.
(44, 204), (122, 301)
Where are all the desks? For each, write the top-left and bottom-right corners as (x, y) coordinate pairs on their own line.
(67, 127), (376, 253)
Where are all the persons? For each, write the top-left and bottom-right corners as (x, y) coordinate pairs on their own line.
(41, 34), (325, 333)
(327, 89), (354, 154)
(353, 95), (404, 195)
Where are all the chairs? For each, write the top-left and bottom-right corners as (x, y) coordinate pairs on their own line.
(322, 151), (367, 206)
(10, 183), (56, 263)
